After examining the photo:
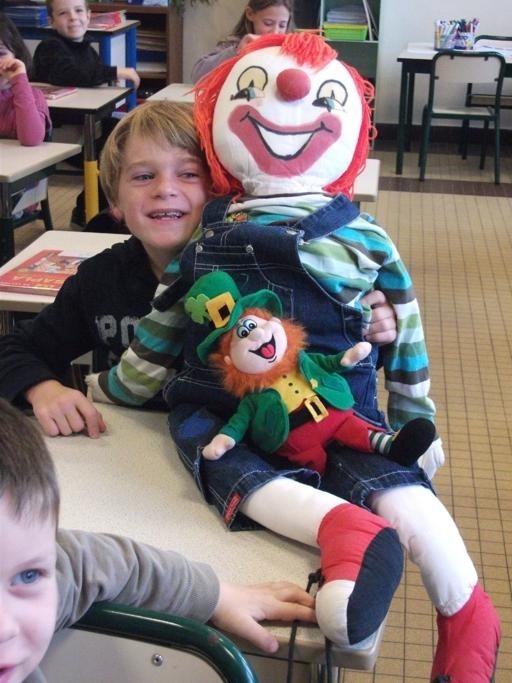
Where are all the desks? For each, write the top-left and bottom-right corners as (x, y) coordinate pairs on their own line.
(13, 18), (142, 113)
(348, 157), (381, 210)
(1, 134), (84, 263)
(33, 399), (389, 683)
(0, 228), (132, 333)
(46, 84), (132, 162)
(147, 81), (197, 105)
(396, 40), (511, 174)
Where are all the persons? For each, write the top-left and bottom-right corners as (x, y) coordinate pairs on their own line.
(84, 33), (502, 683)
(0, 100), (217, 441)
(0, 399), (318, 683)
(33, 0), (141, 159)
(191, 0), (299, 87)
(0, 14), (53, 214)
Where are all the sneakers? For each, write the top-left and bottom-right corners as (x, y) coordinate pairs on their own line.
(306, 502), (401, 647)
(431, 583), (501, 682)
(67, 213), (90, 232)
(390, 416), (437, 467)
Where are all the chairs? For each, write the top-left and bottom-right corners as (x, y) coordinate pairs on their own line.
(40, 600), (258, 683)
(419, 49), (506, 186)
(460, 34), (512, 162)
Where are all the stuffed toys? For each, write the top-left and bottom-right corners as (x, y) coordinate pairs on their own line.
(183, 270), (436, 483)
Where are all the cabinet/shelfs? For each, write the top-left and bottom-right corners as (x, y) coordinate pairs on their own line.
(90, 5), (183, 117)
(292, 1), (381, 150)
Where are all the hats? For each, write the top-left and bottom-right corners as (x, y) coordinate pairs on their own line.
(185, 270), (283, 364)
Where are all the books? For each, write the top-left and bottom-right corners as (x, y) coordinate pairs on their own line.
(323, 0), (378, 41)
(136, 30), (168, 53)
(0, 249), (98, 298)
(4, 6), (48, 28)
(90, 9), (128, 30)
(35, 85), (79, 100)
